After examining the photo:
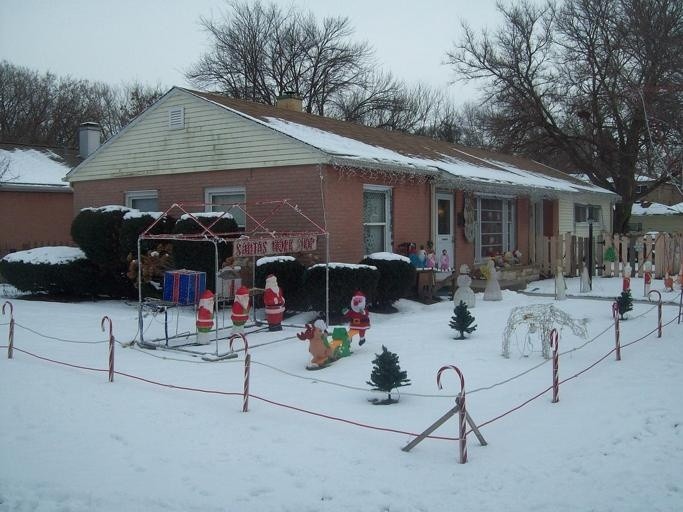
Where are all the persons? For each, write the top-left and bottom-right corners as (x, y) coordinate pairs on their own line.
(231, 285), (252, 327)
(423, 242), (436, 270)
(484, 259), (501, 301)
(622, 264), (632, 292)
(494, 251), (504, 267)
(504, 251), (513, 267)
(195, 288), (216, 333)
(643, 259), (652, 297)
(677, 262), (683, 288)
(440, 248), (450, 272)
(512, 248), (523, 265)
(663, 268), (674, 293)
(263, 273), (287, 332)
